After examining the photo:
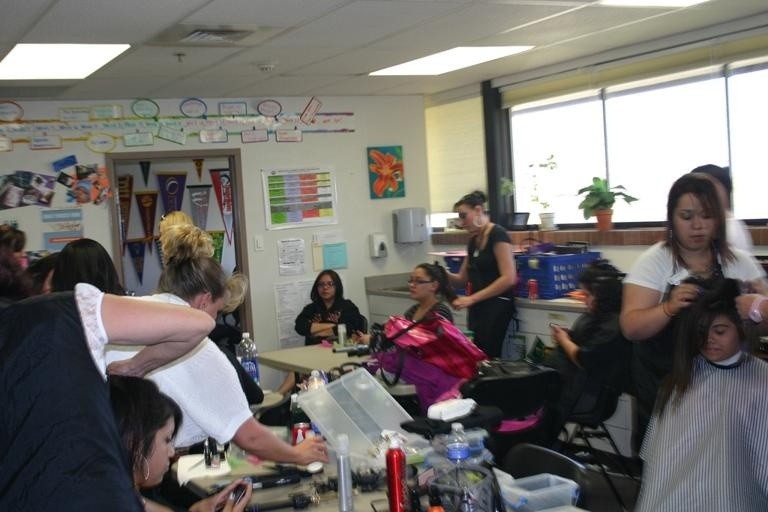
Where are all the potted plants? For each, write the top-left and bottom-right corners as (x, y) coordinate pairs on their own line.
(573, 177), (641, 232)
(497, 176), (532, 230)
(527, 154), (560, 231)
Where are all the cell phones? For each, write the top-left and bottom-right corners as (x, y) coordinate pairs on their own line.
(233, 483), (247, 505)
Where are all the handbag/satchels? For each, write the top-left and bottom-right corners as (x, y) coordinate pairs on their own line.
(384, 311), (486, 381)
(375, 347), (463, 404)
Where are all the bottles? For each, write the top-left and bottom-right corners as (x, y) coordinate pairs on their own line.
(387, 441), (410, 511)
(335, 433), (354, 512)
(307, 371), (328, 435)
(447, 423), (469, 466)
(237, 331), (259, 386)
(338, 323), (347, 345)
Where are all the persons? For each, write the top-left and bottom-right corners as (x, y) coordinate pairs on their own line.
(276, 270), (363, 395)
(403, 262), (458, 324)
(1, 209), (329, 511)
(692, 164), (753, 252)
(634, 278), (768, 512)
(544, 259), (627, 425)
(618, 173), (768, 459)
(734, 293), (768, 324)
(442, 191), (517, 362)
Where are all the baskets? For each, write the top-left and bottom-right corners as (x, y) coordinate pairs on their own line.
(515, 252), (600, 300)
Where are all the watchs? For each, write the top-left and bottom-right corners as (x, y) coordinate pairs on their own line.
(749, 295), (768, 323)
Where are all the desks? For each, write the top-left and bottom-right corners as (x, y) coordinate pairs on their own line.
(170, 424), (594, 512)
(253, 343), (417, 397)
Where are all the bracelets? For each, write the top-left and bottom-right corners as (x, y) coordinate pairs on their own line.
(661, 300), (673, 319)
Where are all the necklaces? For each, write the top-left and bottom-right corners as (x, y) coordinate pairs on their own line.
(472, 229), (485, 258)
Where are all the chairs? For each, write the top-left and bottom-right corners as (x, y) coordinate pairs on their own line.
(460, 368), (561, 444)
(504, 444), (593, 508)
(549, 380), (635, 512)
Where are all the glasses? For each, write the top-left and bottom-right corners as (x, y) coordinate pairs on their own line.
(317, 281), (334, 288)
(408, 279), (434, 286)
